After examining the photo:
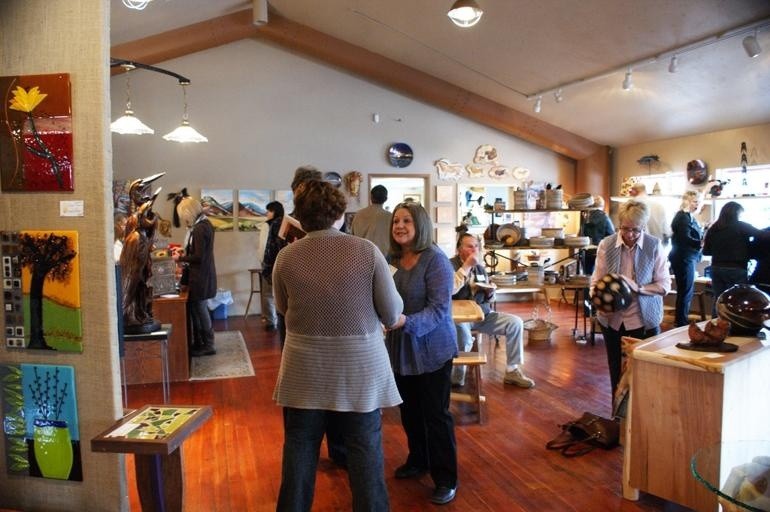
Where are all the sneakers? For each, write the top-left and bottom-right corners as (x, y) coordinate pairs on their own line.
(504, 369), (535, 388)
(451, 372), (465, 387)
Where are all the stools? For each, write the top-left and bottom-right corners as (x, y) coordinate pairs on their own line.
(452, 352), (489, 425)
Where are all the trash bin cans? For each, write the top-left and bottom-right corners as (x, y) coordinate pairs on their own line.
(208, 288), (233, 319)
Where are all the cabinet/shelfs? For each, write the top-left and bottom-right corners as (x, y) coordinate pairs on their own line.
(488, 208), (596, 343)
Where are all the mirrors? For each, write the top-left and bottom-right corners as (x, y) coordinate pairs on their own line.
(367, 173), (432, 235)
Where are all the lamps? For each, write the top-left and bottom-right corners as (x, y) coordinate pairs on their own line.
(526, 16), (770, 120)
(448, 0), (485, 28)
(110, 57), (210, 144)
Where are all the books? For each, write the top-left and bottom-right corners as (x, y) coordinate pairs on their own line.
(278, 214), (308, 242)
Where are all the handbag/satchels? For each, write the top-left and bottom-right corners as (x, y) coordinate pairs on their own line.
(180, 268), (190, 285)
(546, 412), (620, 458)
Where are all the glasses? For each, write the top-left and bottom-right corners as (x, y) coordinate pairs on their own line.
(619, 224), (645, 233)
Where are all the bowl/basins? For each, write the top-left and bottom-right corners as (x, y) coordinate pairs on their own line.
(529, 236), (556, 248)
(569, 275), (592, 284)
(541, 227), (562, 239)
(496, 224), (522, 246)
(571, 192), (598, 209)
(564, 236), (591, 247)
(491, 275), (517, 287)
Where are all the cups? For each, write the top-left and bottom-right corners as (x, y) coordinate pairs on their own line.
(527, 266), (544, 286)
(514, 190), (527, 210)
(495, 201), (505, 212)
(547, 190), (562, 210)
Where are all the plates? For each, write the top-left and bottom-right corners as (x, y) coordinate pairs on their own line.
(475, 282), (496, 289)
(486, 243), (503, 249)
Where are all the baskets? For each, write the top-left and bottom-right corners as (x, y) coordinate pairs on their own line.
(523, 302), (559, 340)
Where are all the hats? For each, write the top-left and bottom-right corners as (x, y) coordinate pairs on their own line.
(291, 164), (323, 190)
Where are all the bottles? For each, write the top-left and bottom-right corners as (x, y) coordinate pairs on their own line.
(549, 274), (556, 284)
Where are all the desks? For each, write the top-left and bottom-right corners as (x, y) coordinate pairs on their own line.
(243, 267), (264, 319)
(123, 324), (173, 409)
(622, 315), (769, 511)
(119, 273), (192, 386)
(449, 299), (487, 393)
(689, 439), (770, 512)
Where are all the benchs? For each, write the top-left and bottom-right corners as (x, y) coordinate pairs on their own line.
(491, 285), (552, 307)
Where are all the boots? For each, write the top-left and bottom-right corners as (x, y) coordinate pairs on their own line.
(193, 328), (202, 351)
(191, 328), (216, 356)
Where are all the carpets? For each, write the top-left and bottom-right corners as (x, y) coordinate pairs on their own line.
(190, 329), (256, 382)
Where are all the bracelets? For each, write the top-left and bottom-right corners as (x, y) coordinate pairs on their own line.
(637, 283), (644, 296)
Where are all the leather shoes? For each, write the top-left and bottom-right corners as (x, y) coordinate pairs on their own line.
(431, 481), (458, 505)
(395, 453), (430, 481)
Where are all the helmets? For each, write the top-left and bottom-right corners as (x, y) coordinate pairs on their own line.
(591, 273), (633, 311)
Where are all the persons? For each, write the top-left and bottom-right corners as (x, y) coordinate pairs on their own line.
(586, 199), (674, 425)
(262, 201), (286, 348)
(166, 196), (220, 357)
(112, 211), (128, 264)
(445, 232), (536, 390)
(745, 224), (770, 308)
(350, 185), (394, 263)
(291, 166), (351, 234)
(380, 201), (458, 505)
(269, 179), (406, 511)
(578, 194), (618, 319)
(666, 189), (711, 327)
(698, 201), (770, 321)
